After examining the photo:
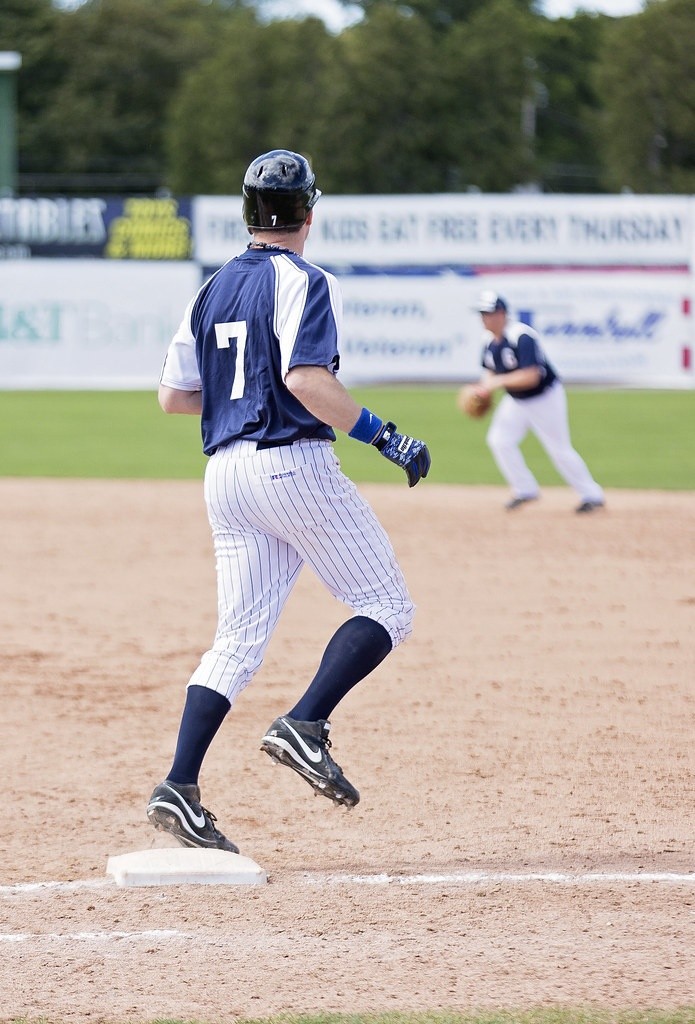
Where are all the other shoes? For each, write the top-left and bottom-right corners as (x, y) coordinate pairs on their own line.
(503, 493), (542, 512)
(572, 499), (605, 516)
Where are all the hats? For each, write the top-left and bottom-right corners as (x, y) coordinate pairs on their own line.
(475, 291), (507, 313)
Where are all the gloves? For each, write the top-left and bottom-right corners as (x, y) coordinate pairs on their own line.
(372, 420), (432, 488)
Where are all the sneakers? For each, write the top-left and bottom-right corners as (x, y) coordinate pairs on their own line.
(147, 780), (241, 854)
(258, 714), (362, 812)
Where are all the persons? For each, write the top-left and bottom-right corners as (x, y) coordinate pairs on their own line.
(463, 289), (605, 512)
(146, 149), (435, 854)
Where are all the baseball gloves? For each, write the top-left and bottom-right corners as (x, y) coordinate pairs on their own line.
(461, 385), (490, 418)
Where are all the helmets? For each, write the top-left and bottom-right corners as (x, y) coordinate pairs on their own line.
(239, 150), (323, 230)
